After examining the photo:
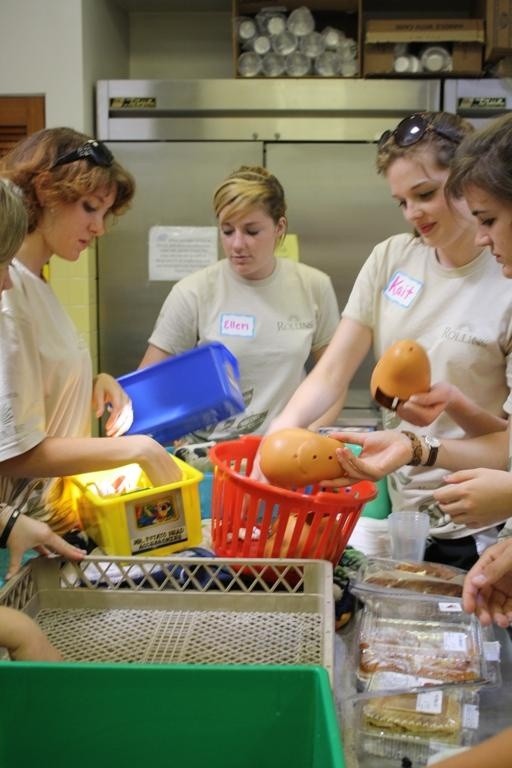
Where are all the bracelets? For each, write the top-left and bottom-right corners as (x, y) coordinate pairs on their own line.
(400, 430), (422, 466)
(0, 508), (20, 549)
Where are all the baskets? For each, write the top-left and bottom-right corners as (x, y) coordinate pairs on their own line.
(209, 434), (379, 584)
(0, 553), (336, 690)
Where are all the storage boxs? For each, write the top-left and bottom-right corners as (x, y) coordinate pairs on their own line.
(230, 2), (365, 79)
(361, 14), (487, 81)
(106, 341), (246, 444)
(2, 661), (345, 767)
(66, 451), (203, 567)
(483, 1), (512, 66)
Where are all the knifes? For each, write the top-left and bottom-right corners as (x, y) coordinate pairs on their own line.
(343, 676), (492, 703)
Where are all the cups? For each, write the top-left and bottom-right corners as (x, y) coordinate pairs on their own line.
(238, 4), (359, 78)
(387, 509), (432, 565)
(392, 41), (452, 72)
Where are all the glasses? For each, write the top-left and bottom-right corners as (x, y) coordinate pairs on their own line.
(373, 116), (461, 148)
(28, 139), (116, 181)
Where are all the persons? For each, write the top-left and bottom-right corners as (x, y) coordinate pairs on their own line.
(0, 126), (183, 554)
(425, 537), (512, 768)
(239, 112), (511, 554)
(318, 108), (511, 542)
(0, 175), (87, 664)
(135, 164), (348, 448)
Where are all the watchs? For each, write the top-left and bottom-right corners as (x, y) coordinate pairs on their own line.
(422, 434), (441, 467)
